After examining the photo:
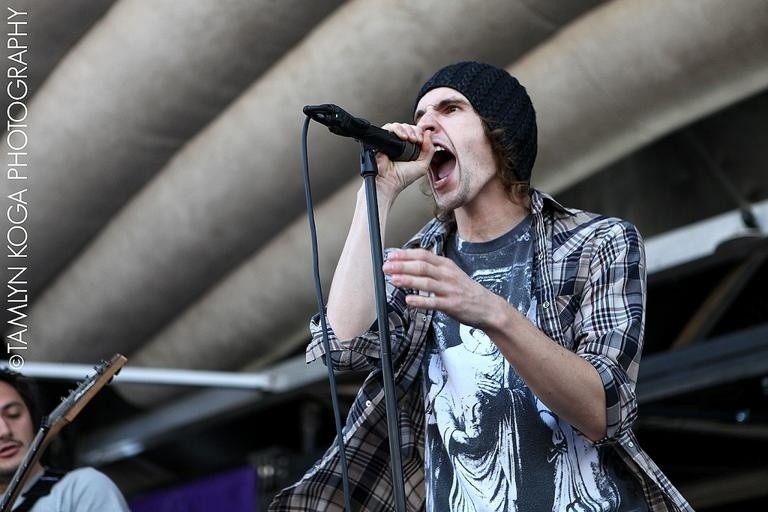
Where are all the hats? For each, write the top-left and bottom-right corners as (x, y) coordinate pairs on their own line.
(413, 61), (537, 185)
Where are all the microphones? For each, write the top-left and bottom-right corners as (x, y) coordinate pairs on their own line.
(302, 101), (422, 163)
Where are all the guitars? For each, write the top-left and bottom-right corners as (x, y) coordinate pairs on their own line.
(1, 351), (126, 512)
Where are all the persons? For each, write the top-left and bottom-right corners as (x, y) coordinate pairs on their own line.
(0, 368), (133, 511)
(265, 60), (695, 510)
